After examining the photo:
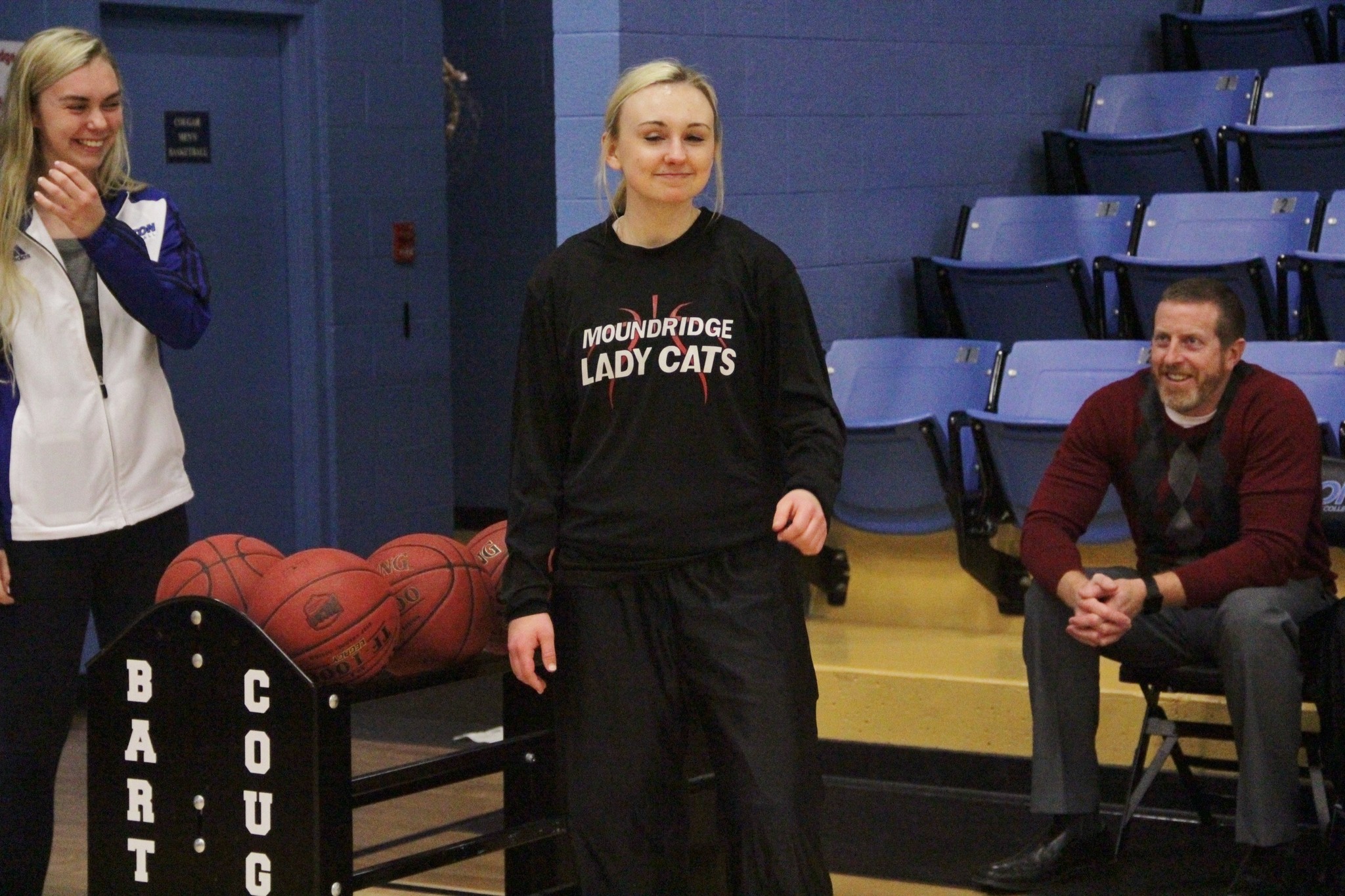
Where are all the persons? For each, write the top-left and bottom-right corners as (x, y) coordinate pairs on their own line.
(0, 26), (210, 896)
(971, 279), (1341, 896)
(502, 59), (845, 896)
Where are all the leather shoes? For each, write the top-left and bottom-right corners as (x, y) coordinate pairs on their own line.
(1224, 864), (1300, 895)
(971, 829), (1108, 892)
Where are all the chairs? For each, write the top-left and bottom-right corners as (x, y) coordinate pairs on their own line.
(1111, 573), (1345, 858)
(821, 0), (1345, 541)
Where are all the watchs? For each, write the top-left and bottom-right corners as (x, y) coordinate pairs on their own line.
(1139, 575), (1163, 617)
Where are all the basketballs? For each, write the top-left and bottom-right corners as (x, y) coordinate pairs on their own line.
(249, 544), (403, 685)
(362, 532), (497, 680)
(466, 520), (554, 660)
(154, 531), (285, 620)
